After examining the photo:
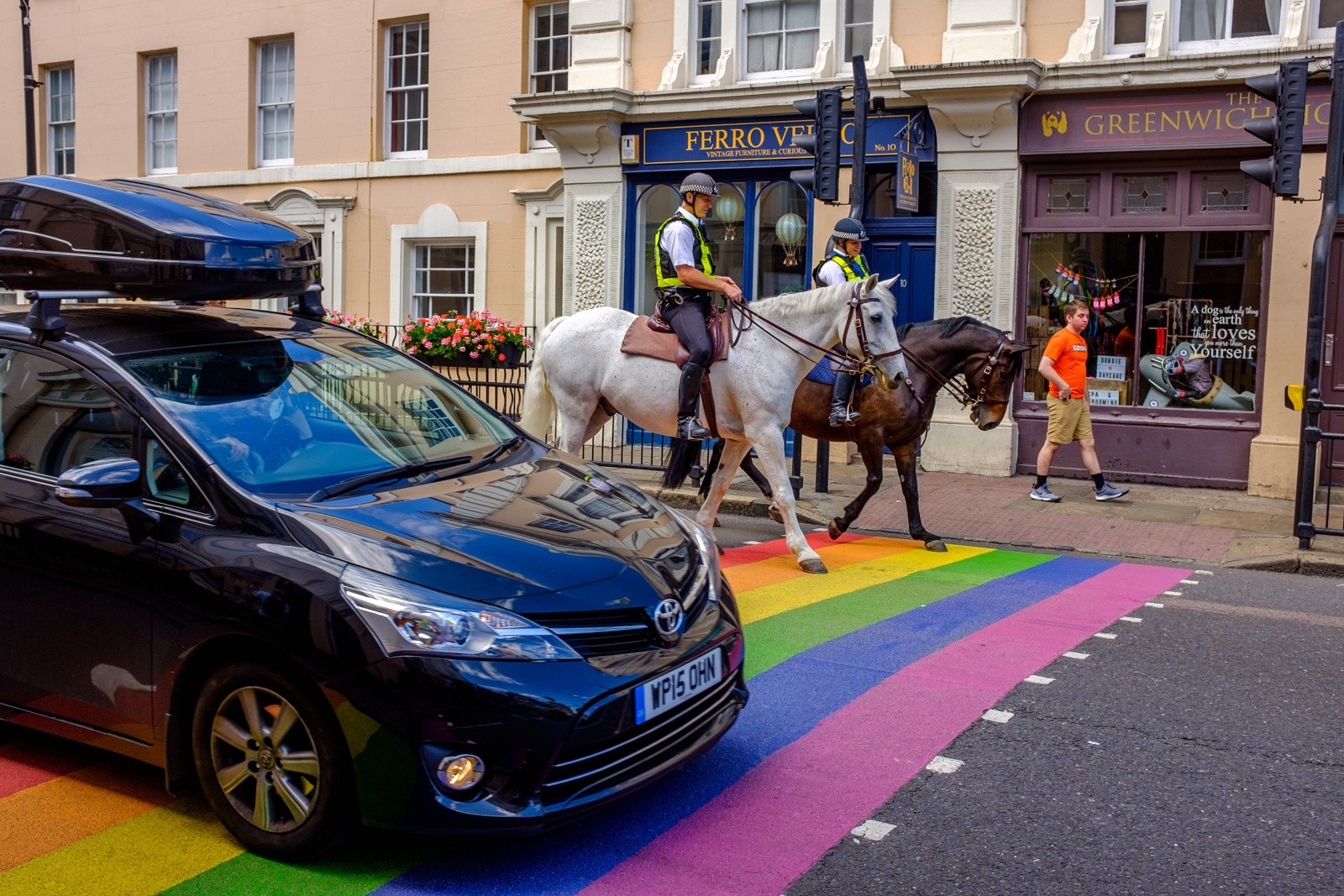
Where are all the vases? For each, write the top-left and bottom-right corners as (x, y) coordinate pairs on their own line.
(413, 341), (523, 369)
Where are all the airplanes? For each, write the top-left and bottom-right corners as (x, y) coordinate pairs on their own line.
(1138, 340), (1256, 411)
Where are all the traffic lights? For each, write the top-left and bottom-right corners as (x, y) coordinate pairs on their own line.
(789, 88), (840, 201)
(1239, 60), (1308, 197)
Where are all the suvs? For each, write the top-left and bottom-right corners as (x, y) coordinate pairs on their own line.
(1, 176), (750, 868)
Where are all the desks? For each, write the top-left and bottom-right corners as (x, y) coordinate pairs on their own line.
(1087, 378), (1133, 405)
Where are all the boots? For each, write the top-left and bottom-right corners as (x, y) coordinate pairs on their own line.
(676, 362), (712, 438)
(829, 365), (862, 427)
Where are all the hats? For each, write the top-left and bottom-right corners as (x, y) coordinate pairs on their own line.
(833, 217), (870, 242)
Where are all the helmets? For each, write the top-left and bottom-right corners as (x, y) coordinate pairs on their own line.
(680, 172), (722, 199)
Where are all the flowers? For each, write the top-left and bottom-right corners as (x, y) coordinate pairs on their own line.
(398, 308), (533, 362)
(322, 309), (386, 341)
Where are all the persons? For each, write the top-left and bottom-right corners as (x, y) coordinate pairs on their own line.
(812, 217), (870, 429)
(652, 171), (742, 439)
(1028, 299), (1130, 503)
(1114, 303), (1148, 376)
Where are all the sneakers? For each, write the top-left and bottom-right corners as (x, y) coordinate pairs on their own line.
(1030, 482), (1062, 502)
(1095, 480), (1129, 500)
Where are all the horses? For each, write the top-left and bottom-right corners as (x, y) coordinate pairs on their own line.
(651, 313), (1037, 554)
(511, 269), (912, 576)
